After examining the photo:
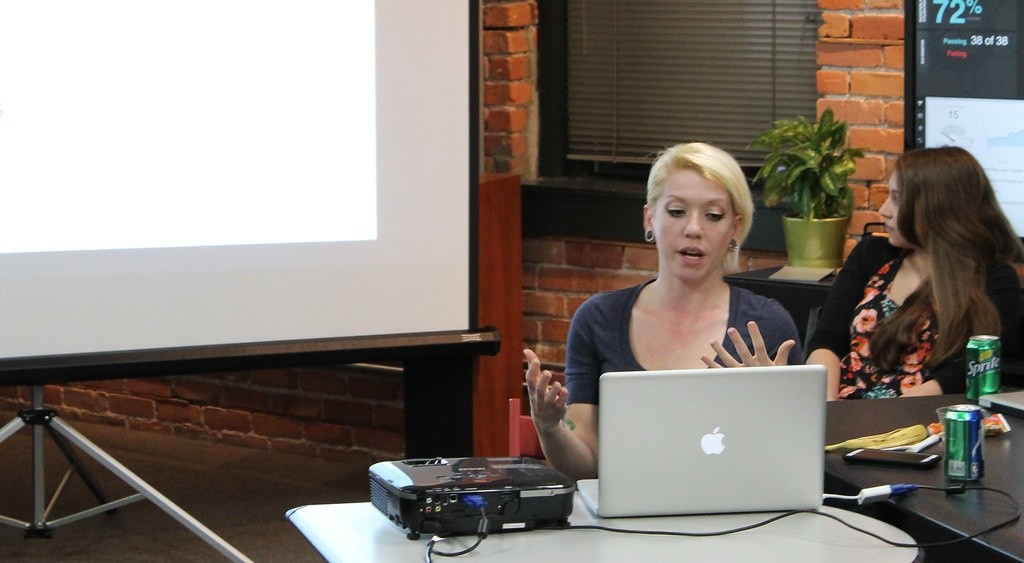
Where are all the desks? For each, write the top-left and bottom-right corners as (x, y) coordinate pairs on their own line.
(286, 475), (923, 563)
(722, 264), (846, 352)
(823, 389), (1023, 561)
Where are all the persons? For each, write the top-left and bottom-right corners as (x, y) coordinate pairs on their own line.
(805, 145), (1024, 400)
(524, 141), (805, 531)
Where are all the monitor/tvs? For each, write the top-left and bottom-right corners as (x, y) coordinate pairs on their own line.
(904, 1), (1023, 263)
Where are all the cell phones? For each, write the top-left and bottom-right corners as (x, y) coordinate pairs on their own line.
(842, 447), (935, 469)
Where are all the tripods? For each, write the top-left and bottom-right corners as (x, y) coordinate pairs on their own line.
(1, 386), (258, 563)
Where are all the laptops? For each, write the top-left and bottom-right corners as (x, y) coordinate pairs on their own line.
(576, 365), (827, 518)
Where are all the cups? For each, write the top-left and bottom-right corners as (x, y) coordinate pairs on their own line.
(936, 406), (946, 448)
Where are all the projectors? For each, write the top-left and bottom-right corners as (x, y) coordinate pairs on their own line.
(368, 457), (575, 539)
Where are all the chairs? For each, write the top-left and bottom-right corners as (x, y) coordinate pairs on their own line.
(506, 398), (546, 462)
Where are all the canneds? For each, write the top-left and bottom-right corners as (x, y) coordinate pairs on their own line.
(943, 334), (1002, 481)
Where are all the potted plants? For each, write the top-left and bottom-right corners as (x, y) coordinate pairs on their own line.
(744, 107), (868, 267)
